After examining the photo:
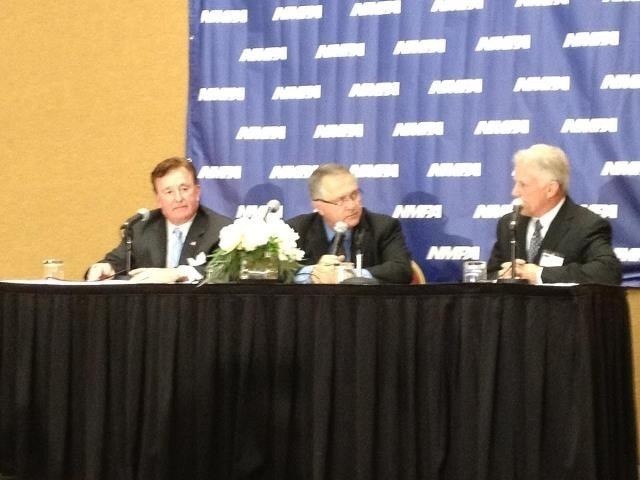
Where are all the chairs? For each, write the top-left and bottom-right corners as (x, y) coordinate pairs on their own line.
(410, 262), (426, 285)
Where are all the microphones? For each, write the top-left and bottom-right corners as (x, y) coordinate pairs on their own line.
(121, 208), (150, 229)
(508, 199), (523, 229)
(264, 199), (282, 220)
(331, 221), (348, 255)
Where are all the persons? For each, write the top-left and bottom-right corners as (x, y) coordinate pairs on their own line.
(84, 155), (234, 287)
(281, 162), (413, 286)
(484, 142), (623, 287)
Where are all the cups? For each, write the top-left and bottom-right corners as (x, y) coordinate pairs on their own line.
(42, 257), (64, 282)
(462, 260), (488, 284)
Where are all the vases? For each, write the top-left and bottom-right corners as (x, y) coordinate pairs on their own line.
(236, 246), (280, 282)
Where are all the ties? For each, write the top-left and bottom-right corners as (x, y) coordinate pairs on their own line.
(526, 219), (543, 262)
(168, 230), (184, 267)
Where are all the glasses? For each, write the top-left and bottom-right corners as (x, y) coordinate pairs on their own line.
(311, 187), (366, 208)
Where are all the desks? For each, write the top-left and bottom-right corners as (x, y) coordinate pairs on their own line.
(0, 279), (638, 480)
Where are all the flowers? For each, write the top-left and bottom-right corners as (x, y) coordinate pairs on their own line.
(203, 213), (308, 284)
